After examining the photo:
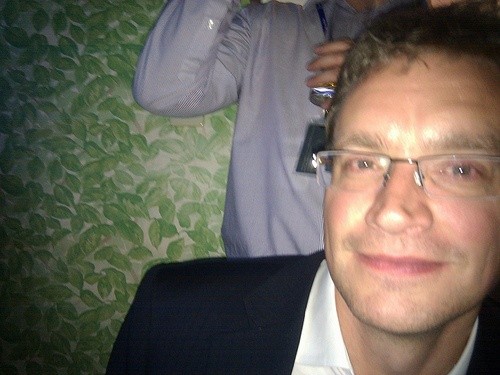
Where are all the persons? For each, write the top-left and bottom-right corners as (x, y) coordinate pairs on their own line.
(132, 0), (397, 257)
(105, 4), (500, 375)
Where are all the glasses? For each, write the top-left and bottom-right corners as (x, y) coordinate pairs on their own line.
(313, 150), (500, 202)
